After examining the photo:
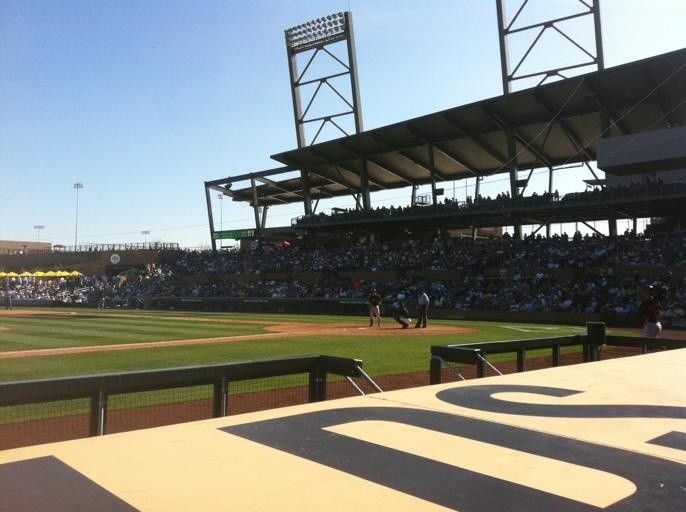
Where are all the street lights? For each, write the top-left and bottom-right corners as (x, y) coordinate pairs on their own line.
(73, 183), (84, 245)
(33, 226), (45, 241)
(217, 192), (224, 246)
(140, 230), (150, 243)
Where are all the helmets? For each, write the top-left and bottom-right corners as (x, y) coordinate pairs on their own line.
(644, 285), (656, 295)
(371, 288), (377, 292)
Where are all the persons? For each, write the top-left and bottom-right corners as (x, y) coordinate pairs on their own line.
(636, 281), (665, 354)
(431, 173), (686, 319)
(368, 288), (383, 328)
(0, 201), (431, 310)
(392, 302), (412, 329)
(415, 288), (430, 328)
